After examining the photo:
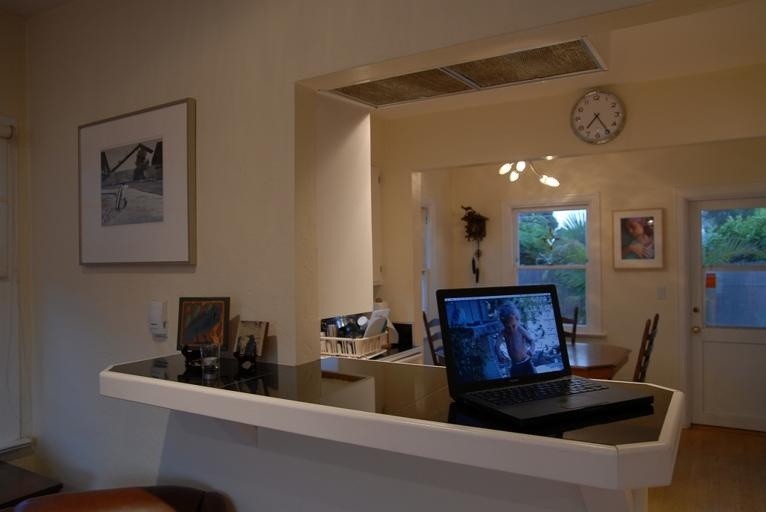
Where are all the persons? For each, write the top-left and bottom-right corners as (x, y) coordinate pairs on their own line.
(493, 302), (541, 378)
(623, 217), (654, 259)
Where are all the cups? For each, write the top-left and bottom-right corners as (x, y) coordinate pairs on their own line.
(198, 343), (222, 379)
(333, 318), (363, 354)
(327, 324), (338, 355)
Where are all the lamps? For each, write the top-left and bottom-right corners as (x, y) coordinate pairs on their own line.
(498, 162), (559, 189)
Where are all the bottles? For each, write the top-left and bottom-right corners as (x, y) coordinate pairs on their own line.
(242, 335), (259, 370)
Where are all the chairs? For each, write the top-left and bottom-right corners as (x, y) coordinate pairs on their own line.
(421, 306), (661, 384)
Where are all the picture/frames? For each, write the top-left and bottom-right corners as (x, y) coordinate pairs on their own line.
(610, 207), (667, 272)
(76, 95), (199, 270)
(174, 294), (231, 352)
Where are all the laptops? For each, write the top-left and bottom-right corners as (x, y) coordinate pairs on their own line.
(435, 282), (655, 427)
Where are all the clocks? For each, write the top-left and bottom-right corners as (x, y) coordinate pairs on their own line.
(569, 87), (627, 146)
